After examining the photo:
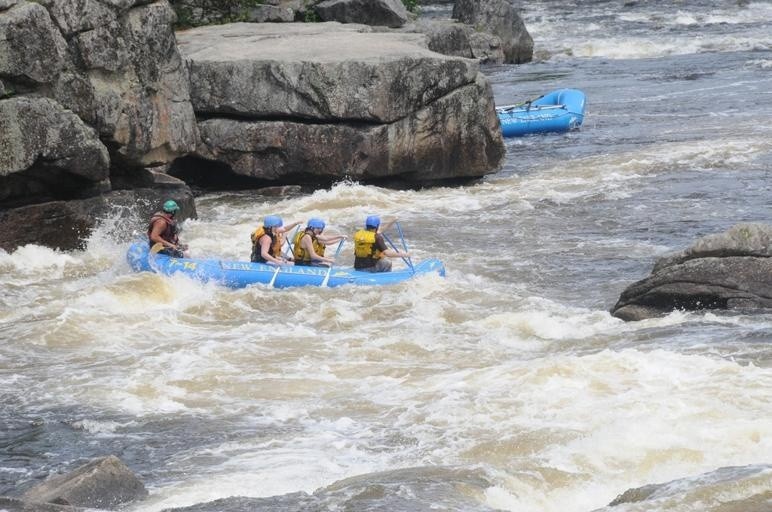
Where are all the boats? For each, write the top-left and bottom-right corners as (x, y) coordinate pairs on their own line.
(127, 242), (445, 290)
(495, 88), (586, 136)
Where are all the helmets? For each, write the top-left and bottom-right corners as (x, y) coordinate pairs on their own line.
(366, 215), (380, 228)
(162, 201), (181, 213)
(308, 219), (325, 229)
(264, 215), (282, 229)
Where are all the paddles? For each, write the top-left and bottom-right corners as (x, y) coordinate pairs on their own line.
(149, 243), (186, 255)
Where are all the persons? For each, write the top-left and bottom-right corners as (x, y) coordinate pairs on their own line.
(148, 200), (188, 257)
(294, 218), (348, 266)
(355, 216), (411, 272)
(250, 216), (304, 266)
